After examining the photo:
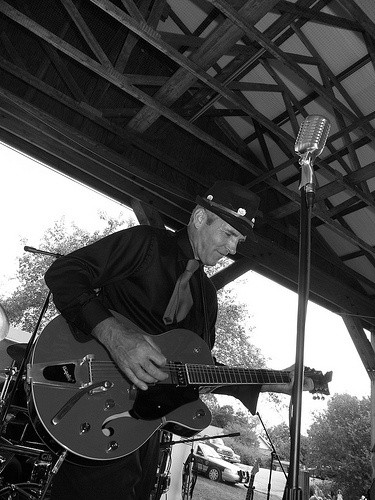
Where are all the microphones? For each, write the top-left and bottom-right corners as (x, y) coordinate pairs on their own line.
(294, 114), (331, 207)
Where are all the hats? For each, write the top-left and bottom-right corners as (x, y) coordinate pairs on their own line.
(196, 180), (259, 244)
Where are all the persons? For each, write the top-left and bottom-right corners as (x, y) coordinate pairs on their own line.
(43, 177), (314, 500)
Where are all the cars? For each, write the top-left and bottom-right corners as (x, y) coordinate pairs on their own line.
(194, 442), (251, 486)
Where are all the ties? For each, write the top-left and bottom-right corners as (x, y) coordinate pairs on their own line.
(161, 260), (200, 325)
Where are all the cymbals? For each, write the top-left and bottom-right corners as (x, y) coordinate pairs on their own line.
(6, 343), (34, 364)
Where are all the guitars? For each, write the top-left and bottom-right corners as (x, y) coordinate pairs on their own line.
(22, 309), (330, 470)
